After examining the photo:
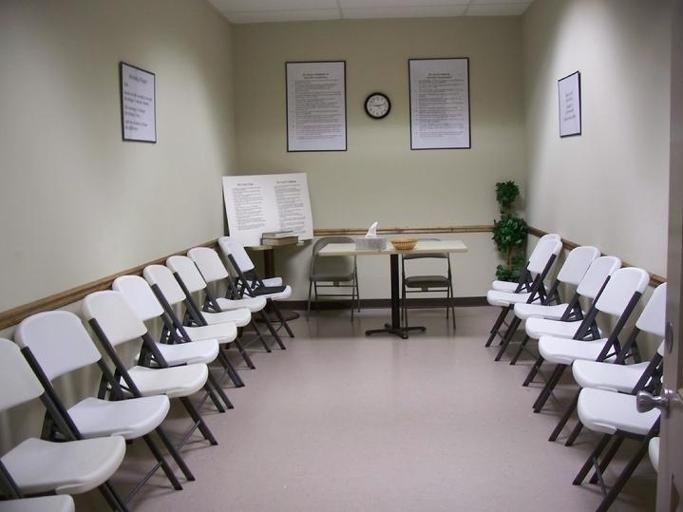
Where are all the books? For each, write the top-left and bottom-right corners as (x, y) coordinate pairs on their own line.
(259, 237), (298, 247)
(262, 229), (298, 238)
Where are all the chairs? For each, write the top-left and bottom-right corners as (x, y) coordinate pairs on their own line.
(398, 236), (456, 329)
(305, 236), (361, 323)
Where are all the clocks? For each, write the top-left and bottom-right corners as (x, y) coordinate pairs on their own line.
(364, 92), (391, 121)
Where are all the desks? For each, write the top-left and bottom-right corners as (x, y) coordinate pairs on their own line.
(319, 239), (469, 340)
(244, 243), (300, 324)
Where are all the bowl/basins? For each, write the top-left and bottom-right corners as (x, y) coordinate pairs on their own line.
(391, 239), (418, 250)
